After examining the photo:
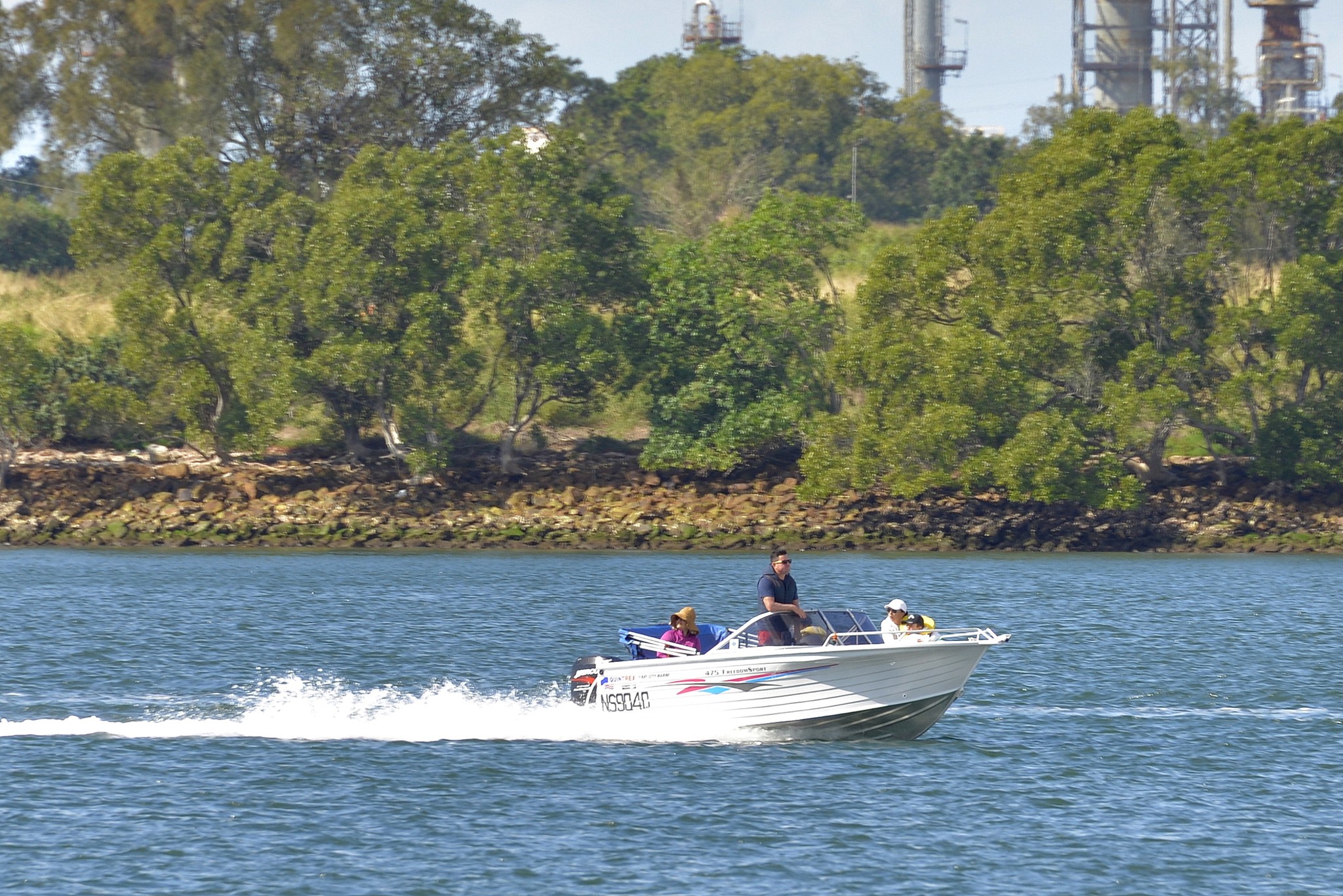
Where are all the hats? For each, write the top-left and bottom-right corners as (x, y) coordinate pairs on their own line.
(671, 607), (700, 635)
(901, 614), (924, 627)
(884, 599), (907, 613)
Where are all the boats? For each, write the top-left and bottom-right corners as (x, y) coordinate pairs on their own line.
(570, 607), (1012, 747)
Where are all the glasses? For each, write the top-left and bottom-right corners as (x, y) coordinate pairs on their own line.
(675, 615), (681, 620)
(772, 559), (792, 566)
(886, 608), (897, 614)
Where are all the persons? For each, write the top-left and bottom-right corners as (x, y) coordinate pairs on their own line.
(790, 614), (813, 646)
(757, 548), (807, 647)
(656, 606), (701, 658)
(880, 598), (942, 644)
